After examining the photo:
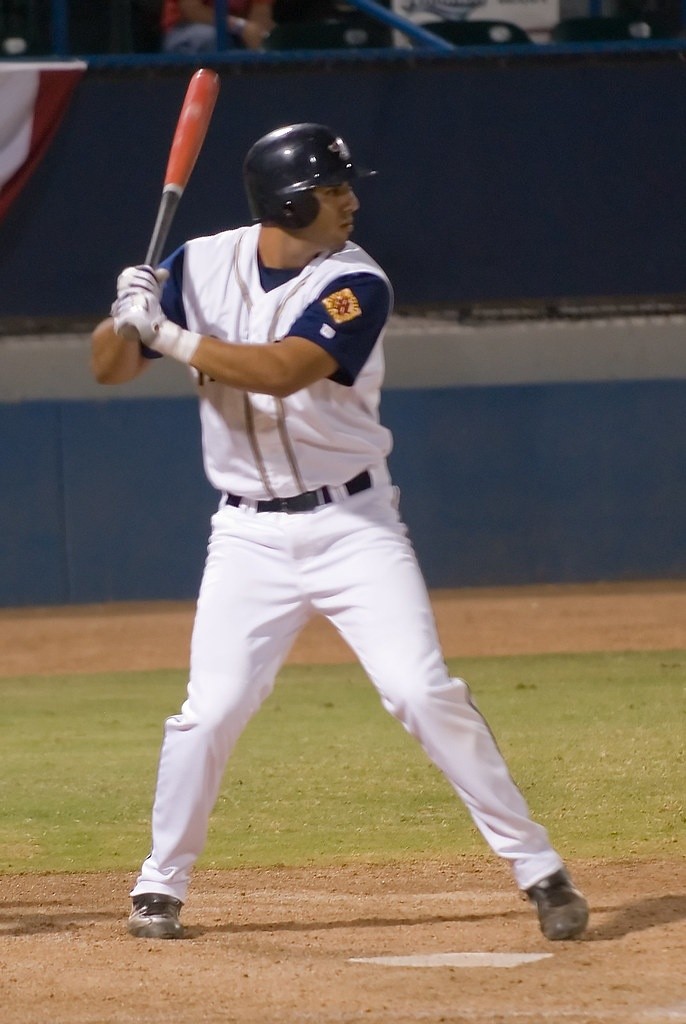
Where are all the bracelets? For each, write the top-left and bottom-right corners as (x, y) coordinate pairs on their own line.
(231, 17), (247, 35)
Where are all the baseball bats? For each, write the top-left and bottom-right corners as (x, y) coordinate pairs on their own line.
(119, 67), (220, 341)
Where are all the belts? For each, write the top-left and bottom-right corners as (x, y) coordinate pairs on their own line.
(226, 471), (372, 511)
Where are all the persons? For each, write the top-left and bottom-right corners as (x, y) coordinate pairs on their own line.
(162, 0), (276, 54)
(89, 122), (591, 942)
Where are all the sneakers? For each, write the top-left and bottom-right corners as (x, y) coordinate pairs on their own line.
(527, 868), (589, 940)
(126, 893), (184, 938)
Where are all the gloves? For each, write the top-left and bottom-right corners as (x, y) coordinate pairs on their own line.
(112, 288), (202, 364)
(116, 264), (170, 302)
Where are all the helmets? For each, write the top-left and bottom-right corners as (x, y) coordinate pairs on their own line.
(243, 123), (380, 231)
